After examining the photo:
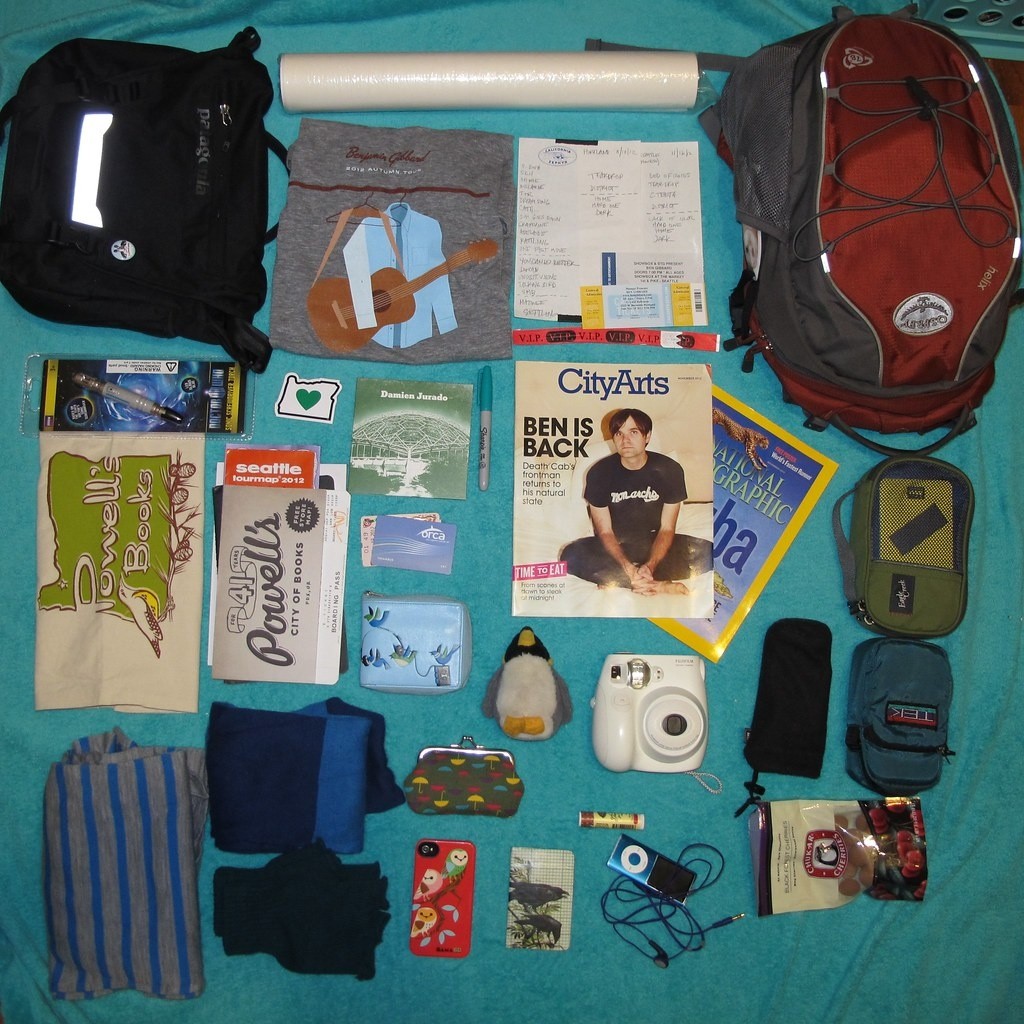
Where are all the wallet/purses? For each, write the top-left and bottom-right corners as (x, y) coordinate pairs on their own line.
(360, 591), (472, 695)
(403, 736), (524, 818)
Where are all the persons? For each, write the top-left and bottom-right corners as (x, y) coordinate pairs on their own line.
(560, 408), (713, 595)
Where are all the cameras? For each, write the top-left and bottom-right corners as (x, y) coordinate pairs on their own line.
(590, 653), (709, 773)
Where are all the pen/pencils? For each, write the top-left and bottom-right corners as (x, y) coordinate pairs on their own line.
(474, 364), (492, 497)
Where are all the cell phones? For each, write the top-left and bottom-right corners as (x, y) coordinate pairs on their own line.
(409, 839), (475, 958)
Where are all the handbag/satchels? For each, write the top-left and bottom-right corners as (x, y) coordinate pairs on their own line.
(0, 27), (291, 374)
(834, 458), (975, 639)
(847, 636), (955, 796)
(735, 617), (832, 817)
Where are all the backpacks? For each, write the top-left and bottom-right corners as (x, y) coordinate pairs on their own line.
(698, 4), (1021, 458)
